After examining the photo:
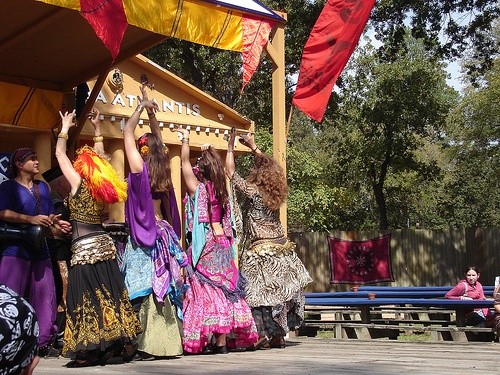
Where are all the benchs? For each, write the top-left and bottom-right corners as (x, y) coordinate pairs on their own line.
(319, 307), (456, 323)
(303, 320), (494, 342)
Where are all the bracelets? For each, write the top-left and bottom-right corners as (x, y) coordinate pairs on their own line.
(228, 147), (233, 151)
(57, 133), (69, 139)
(136, 105), (144, 113)
(92, 136), (103, 142)
(182, 138), (189, 143)
(148, 108), (154, 115)
(253, 147), (258, 152)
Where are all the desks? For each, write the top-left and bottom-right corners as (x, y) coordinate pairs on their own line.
(299, 285), (500, 342)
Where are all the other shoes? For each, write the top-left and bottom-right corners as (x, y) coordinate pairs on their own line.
(39, 344), (62, 358)
(66, 348), (111, 368)
(202, 341), (228, 354)
(270, 337), (286, 348)
(247, 335), (271, 351)
(134, 351), (155, 361)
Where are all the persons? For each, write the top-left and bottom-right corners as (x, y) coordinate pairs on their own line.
(0, 285), (40, 375)
(55, 107), (142, 363)
(225, 128), (313, 348)
(445, 266), (500, 336)
(0, 148), (72, 358)
(173, 124), (258, 354)
(121, 85), (188, 357)
